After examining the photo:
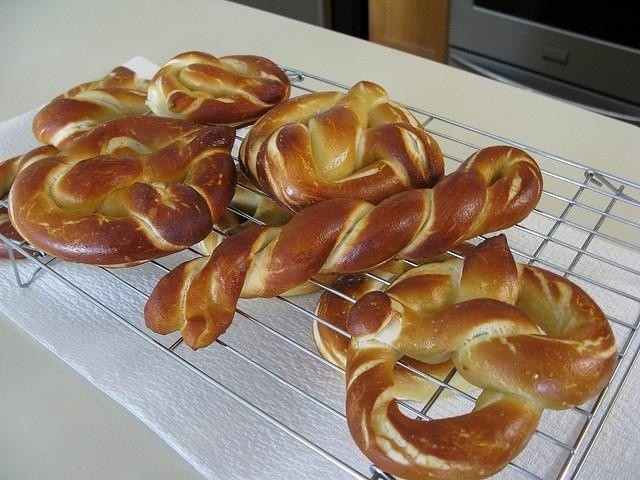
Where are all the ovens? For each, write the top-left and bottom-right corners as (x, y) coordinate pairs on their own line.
(445, 1), (639, 123)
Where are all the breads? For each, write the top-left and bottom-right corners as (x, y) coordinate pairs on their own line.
(33, 65), (155, 147)
(145, 51), (291, 125)
(8, 119), (238, 268)
(195, 163), (351, 297)
(1, 153), (47, 259)
(311, 238), (504, 394)
(144, 145), (543, 349)
(343, 231), (618, 480)
(238, 79), (443, 207)
